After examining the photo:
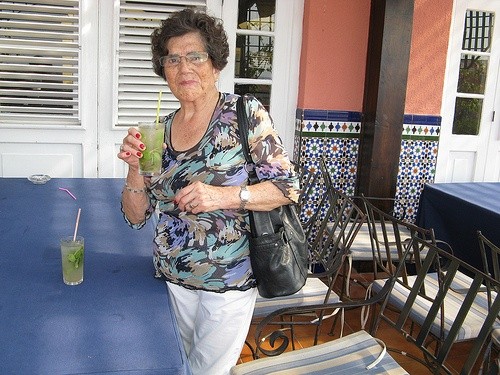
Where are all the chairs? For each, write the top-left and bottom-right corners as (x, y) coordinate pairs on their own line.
(224, 152), (500, 375)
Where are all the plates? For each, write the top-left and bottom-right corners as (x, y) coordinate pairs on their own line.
(27, 174), (50, 185)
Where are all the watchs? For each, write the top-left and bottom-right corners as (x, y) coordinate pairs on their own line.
(238, 184), (250, 211)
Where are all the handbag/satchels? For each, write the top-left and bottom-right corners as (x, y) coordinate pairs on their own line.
(236, 94), (309, 300)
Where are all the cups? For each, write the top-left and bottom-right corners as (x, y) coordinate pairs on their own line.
(139, 121), (165, 175)
(60, 235), (84, 285)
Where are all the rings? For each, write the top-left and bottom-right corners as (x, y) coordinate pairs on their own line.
(120, 145), (124, 150)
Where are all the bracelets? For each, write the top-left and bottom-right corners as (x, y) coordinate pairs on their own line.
(125, 179), (148, 194)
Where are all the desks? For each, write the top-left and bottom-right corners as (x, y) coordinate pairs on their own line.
(414, 182), (500, 294)
(0, 178), (190, 375)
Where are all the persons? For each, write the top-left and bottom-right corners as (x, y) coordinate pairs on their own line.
(28, 55), (66, 107)
(117, 8), (307, 375)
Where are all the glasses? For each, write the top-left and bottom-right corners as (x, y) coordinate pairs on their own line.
(158, 50), (209, 68)
